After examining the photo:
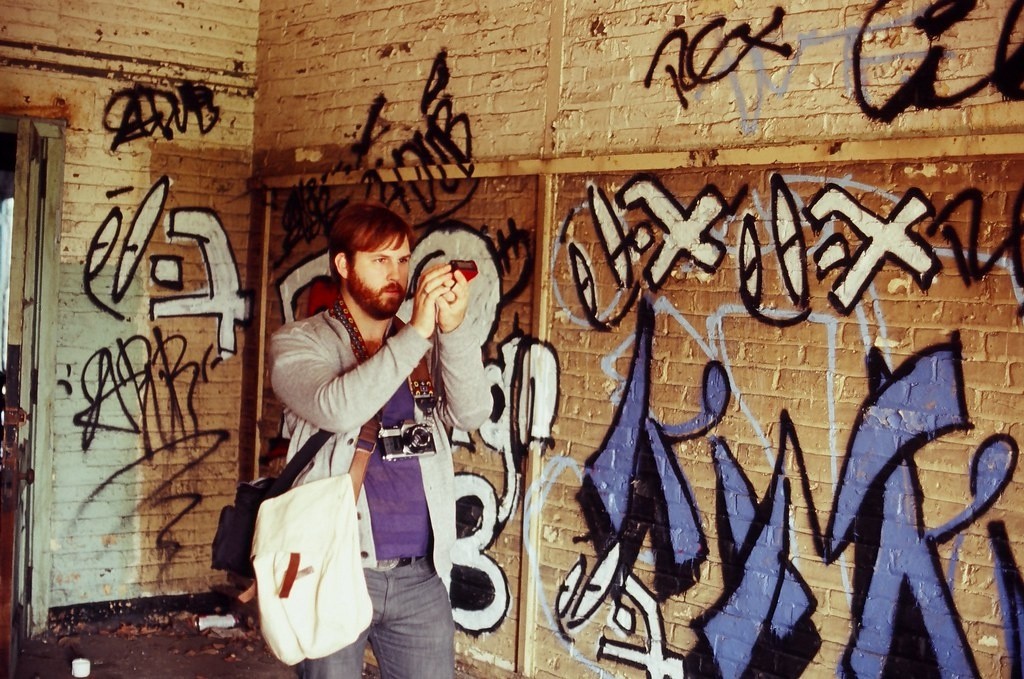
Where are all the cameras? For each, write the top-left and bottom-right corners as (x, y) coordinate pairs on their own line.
(448, 259), (478, 288)
(377, 419), (437, 461)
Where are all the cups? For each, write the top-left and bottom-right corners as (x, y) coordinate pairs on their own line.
(72, 660), (90, 677)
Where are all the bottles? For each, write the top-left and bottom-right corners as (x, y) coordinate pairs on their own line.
(198, 614), (241, 631)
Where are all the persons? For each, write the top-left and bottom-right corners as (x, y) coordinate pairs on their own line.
(269, 204), (494, 679)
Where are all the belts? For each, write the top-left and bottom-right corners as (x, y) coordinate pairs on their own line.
(375, 556), (424, 571)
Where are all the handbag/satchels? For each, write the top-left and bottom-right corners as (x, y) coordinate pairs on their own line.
(249, 473), (374, 666)
(211, 477), (291, 578)
